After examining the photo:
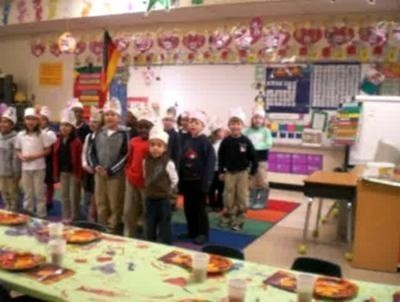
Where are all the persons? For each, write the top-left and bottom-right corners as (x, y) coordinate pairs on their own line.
(1, 97), (212, 247)
(213, 105), (275, 231)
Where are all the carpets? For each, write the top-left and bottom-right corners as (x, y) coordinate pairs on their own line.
(0, 180), (300, 251)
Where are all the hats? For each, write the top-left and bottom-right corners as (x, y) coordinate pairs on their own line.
(2, 105), (49, 125)
(129, 111), (222, 144)
(252, 105), (265, 117)
(227, 107), (246, 126)
(60, 97), (121, 128)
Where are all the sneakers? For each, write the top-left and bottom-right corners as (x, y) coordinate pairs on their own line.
(179, 232), (205, 245)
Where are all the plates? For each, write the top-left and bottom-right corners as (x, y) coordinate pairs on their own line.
(62, 219), (104, 245)
(273, 271), (359, 300)
(1, 251), (45, 273)
(1, 213), (26, 227)
(185, 254), (229, 274)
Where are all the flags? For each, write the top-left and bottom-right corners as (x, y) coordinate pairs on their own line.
(99, 30), (122, 94)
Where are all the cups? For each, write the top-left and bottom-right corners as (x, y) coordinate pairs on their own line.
(47, 222), (65, 239)
(227, 278), (247, 302)
(293, 273), (319, 302)
(190, 251), (211, 285)
(49, 239), (66, 267)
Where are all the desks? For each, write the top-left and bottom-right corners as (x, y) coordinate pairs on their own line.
(300, 170), (357, 249)
(0, 210), (400, 302)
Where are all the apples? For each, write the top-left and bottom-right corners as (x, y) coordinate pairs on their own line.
(298, 245), (306, 253)
(345, 252), (352, 261)
